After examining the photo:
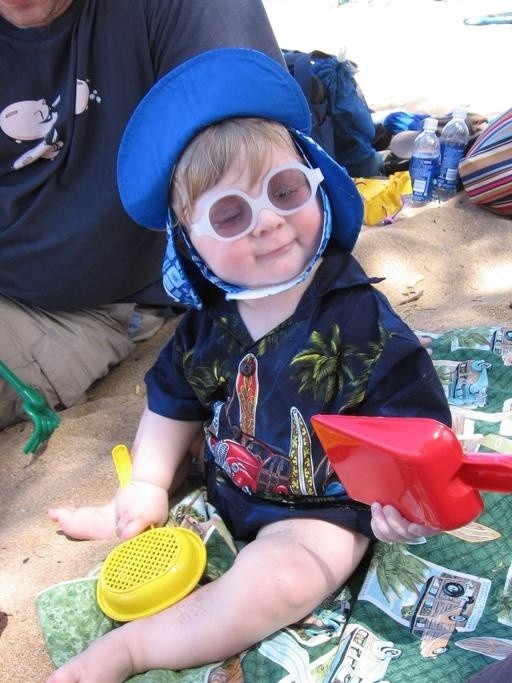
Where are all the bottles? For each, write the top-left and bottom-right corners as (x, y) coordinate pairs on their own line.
(434, 109), (470, 201)
(409, 117), (442, 203)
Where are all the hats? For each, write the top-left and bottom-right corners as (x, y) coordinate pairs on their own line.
(118, 49), (364, 309)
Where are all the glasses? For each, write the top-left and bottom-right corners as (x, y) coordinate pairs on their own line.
(192, 163), (324, 242)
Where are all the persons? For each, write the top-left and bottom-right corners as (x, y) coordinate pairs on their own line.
(0, 1), (286, 431)
(39, 46), (452, 682)
